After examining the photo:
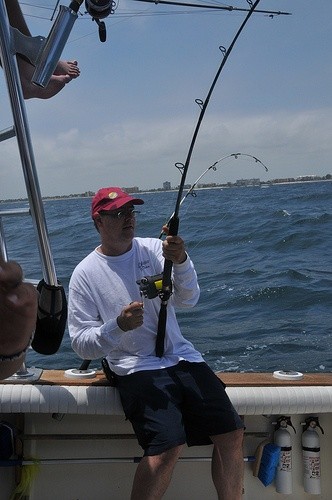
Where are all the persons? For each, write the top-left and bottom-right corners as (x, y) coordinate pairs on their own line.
(67, 187), (247, 500)
(0, 0), (81, 100)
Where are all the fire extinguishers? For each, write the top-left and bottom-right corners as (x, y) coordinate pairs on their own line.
(300, 417), (324, 494)
(271, 416), (296, 494)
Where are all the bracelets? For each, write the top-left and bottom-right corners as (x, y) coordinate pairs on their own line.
(0, 325), (36, 362)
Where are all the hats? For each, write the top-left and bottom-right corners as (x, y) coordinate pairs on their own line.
(92, 188), (144, 219)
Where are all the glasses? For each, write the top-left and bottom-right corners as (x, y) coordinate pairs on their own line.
(100, 209), (141, 219)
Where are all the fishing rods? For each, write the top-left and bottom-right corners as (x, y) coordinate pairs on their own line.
(156, 151), (268, 239)
(138, 0), (264, 356)
